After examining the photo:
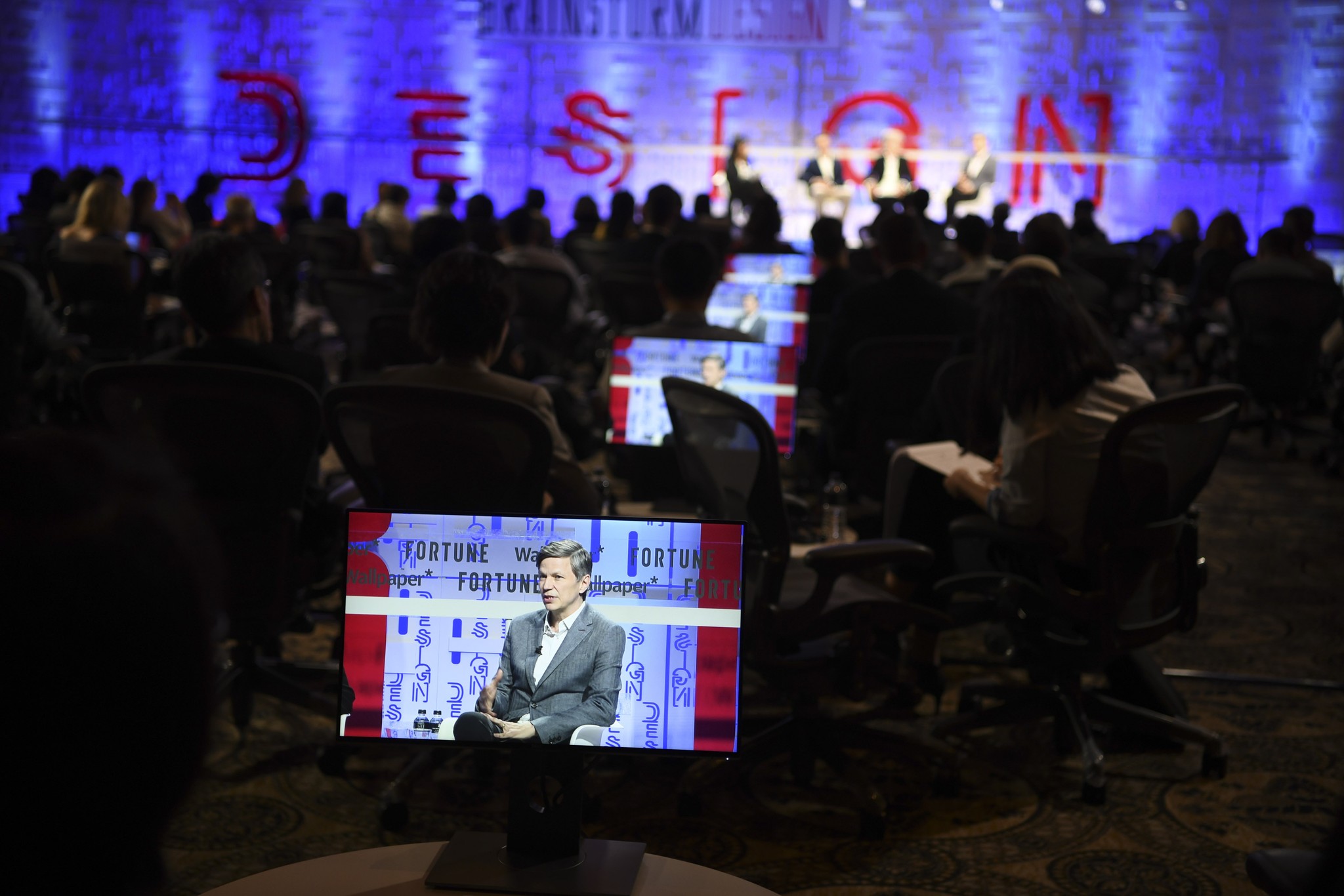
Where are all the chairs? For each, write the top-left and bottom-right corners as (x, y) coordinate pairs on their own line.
(0, 229), (1343, 896)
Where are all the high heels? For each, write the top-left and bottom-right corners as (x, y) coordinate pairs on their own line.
(884, 664), (948, 714)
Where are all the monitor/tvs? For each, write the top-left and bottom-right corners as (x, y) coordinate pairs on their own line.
(601, 332), (798, 458)
(702, 251), (825, 345)
(332, 505), (746, 896)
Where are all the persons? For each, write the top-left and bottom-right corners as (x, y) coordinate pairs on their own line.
(862, 128), (913, 201)
(0, 166), (1344, 718)
(726, 139), (763, 207)
(475, 539), (626, 746)
(946, 133), (996, 219)
(798, 133), (850, 222)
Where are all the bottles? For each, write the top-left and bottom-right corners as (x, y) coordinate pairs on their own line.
(821, 468), (848, 544)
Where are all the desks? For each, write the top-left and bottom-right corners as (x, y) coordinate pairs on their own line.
(201, 840), (781, 896)
